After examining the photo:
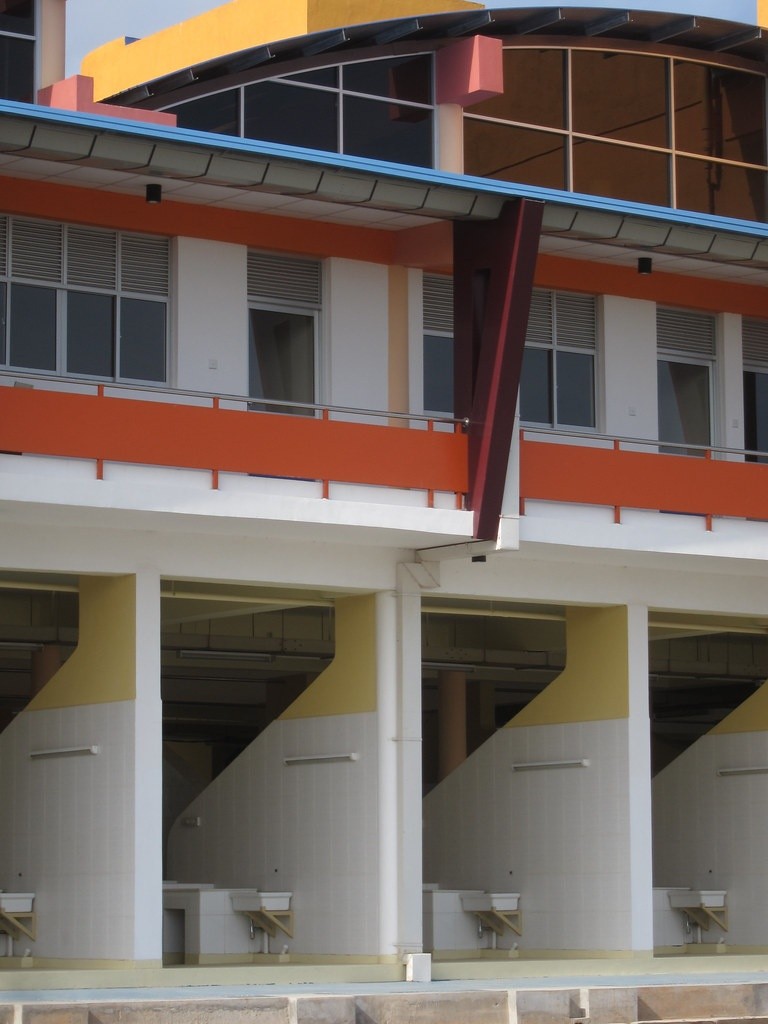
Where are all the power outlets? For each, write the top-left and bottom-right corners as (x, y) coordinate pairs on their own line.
(182, 817), (201, 827)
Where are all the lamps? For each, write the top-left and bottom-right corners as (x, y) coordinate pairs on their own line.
(146, 184), (161, 204)
(716, 767), (768, 777)
(282, 752), (358, 767)
(0, 642), (45, 652)
(512, 758), (590, 771)
(421, 662), (476, 674)
(29, 746), (97, 760)
(638, 257), (652, 275)
(177, 649), (276, 664)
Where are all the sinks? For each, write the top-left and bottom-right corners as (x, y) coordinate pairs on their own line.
(0, 893), (36, 913)
(228, 891), (294, 911)
(459, 893), (521, 911)
(667, 890), (727, 907)
